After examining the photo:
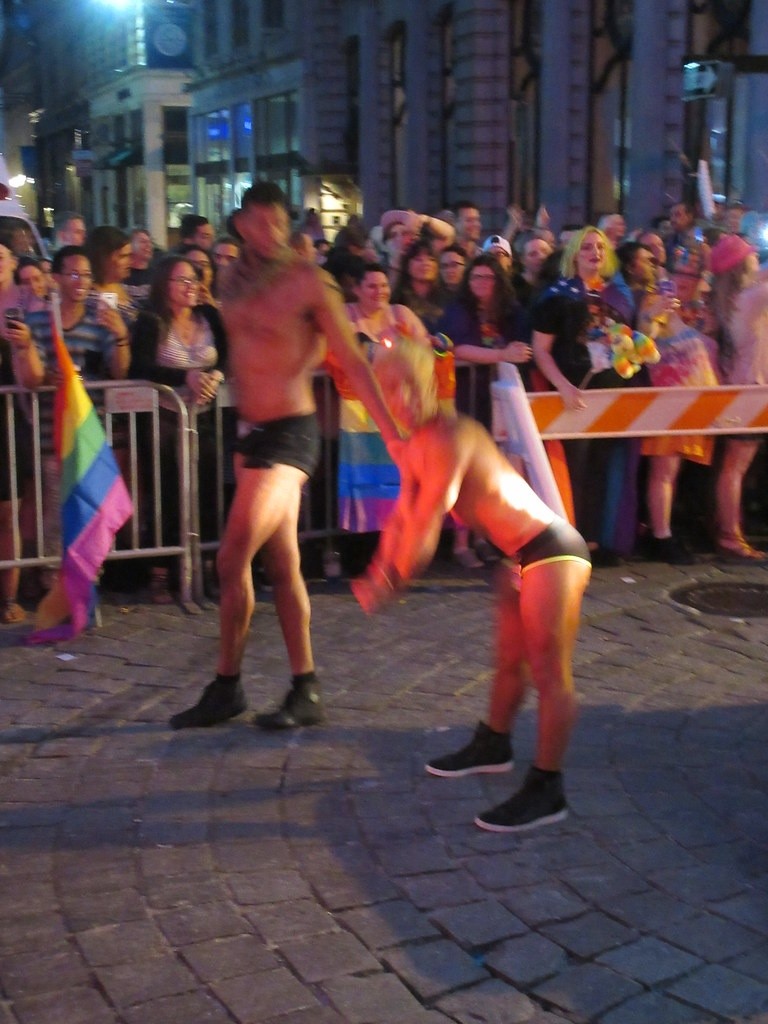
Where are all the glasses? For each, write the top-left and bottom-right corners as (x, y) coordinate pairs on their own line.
(439, 260), (466, 270)
(62, 271), (97, 282)
(168, 275), (201, 286)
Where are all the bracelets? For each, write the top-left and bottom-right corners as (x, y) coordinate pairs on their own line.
(115, 335), (130, 342)
(378, 562), (401, 591)
(114, 343), (131, 347)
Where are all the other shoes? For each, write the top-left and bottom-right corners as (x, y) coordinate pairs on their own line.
(642, 533), (696, 566)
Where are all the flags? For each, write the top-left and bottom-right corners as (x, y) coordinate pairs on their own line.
(29, 311), (134, 643)
(337, 355), (454, 533)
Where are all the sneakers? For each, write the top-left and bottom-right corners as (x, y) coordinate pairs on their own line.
(169, 681), (249, 729)
(424, 719), (515, 778)
(474, 764), (569, 833)
(254, 681), (328, 731)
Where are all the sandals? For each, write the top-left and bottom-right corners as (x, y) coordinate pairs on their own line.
(715, 532), (767, 565)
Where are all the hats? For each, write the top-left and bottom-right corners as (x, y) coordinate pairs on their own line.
(709, 234), (755, 273)
(728, 196), (750, 212)
(481, 234), (513, 258)
(380, 209), (413, 227)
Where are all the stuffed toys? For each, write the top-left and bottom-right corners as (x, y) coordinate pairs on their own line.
(589, 318), (661, 378)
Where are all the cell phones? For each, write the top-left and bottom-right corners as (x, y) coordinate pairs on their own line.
(661, 280), (676, 296)
(6, 307), (19, 329)
(98, 293), (118, 314)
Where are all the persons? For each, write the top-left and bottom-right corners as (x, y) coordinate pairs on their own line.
(0, 209), (245, 623)
(352, 338), (592, 834)
(291, 202), (768, 563)
(169, 182), (404, 732)
